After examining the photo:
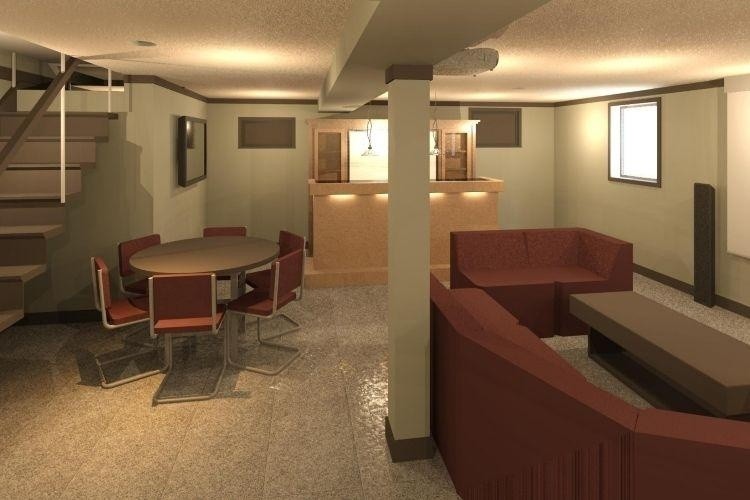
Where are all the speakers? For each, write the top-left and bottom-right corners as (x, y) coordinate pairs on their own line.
(693, 182), (716, 308)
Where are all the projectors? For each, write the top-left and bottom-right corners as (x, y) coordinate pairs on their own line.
(433, 48), (500, 75)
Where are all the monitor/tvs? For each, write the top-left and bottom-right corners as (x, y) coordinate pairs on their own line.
(178, 115), (208, 188)
